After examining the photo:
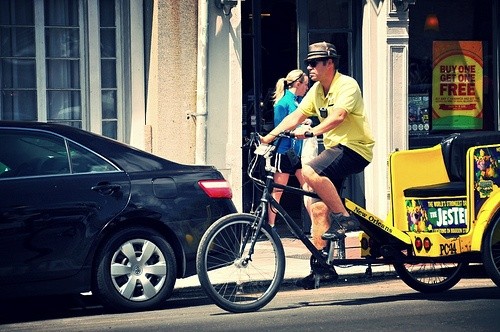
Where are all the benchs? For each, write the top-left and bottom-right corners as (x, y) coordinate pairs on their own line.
(14, 156), (70, 175)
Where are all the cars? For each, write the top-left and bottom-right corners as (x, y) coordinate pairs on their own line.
(1, 121), (241, 316)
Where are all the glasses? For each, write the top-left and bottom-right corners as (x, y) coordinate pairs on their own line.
(304, 59), (319, 67)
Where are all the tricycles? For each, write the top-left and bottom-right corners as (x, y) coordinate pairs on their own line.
(196, 129), (500, 313)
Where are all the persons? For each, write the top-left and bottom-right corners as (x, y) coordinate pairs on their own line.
(268, 69), (314, 238)
(258, 41), (375, 290)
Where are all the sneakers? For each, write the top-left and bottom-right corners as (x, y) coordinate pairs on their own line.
(296, 271), (338, 286)
(321, 213), (360, 239)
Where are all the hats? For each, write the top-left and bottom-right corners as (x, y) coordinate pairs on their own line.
(307, 42), (340, 59)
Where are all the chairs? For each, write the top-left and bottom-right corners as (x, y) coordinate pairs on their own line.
(388, 131), (500, 233)
